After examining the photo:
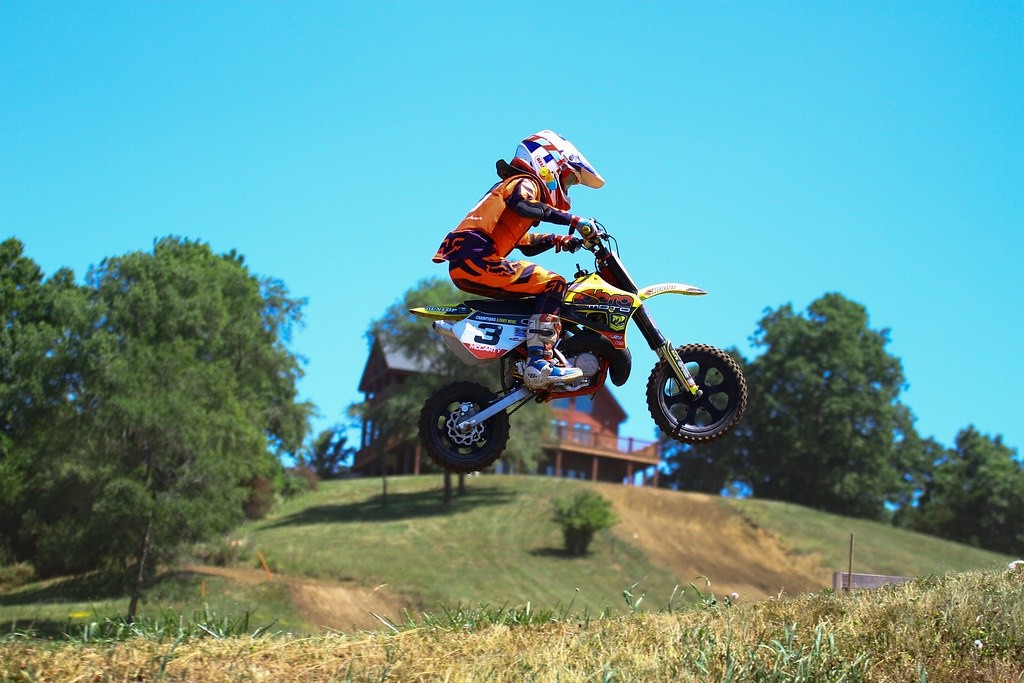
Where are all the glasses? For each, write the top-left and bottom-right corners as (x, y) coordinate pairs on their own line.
(562, 168), (576, 191)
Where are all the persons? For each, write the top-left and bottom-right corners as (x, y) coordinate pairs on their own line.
(432, 129), (605, 387)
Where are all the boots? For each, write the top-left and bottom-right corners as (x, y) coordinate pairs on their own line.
(523, 313), (584, 390)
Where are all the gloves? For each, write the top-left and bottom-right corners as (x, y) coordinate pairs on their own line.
(569, 216), (603, 241)
(552, 235), (582, 254)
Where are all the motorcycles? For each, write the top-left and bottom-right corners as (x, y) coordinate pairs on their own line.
(409, 221), (748, 472)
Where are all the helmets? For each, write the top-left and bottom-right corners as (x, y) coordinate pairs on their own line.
(510, 129), (606, 211)
